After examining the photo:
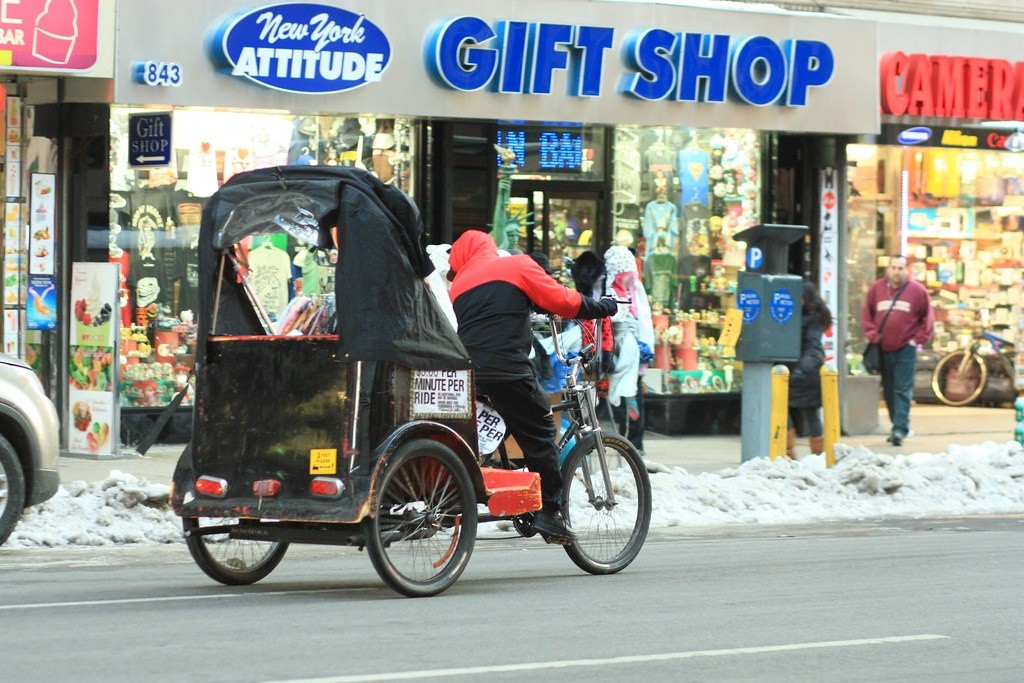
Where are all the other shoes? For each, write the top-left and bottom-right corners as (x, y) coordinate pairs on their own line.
(892, 435), (902, 445)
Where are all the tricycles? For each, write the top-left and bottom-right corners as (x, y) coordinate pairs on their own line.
(170, 165), (653, 599)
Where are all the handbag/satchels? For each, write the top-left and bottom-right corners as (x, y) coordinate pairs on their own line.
(863, 344), (880, 374)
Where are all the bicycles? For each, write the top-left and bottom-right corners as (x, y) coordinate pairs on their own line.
(931, 309), (1024, 408)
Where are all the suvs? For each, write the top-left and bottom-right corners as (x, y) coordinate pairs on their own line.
(0, 352), (62, 548)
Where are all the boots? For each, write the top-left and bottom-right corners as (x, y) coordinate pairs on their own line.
(786, 429), (795, 462)
(809, 434), (823, 456)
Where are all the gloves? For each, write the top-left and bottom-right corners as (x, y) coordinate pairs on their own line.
(601, 298), (618, 317)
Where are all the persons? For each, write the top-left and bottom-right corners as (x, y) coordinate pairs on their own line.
(788, 282), (833, 461)
(486, 174), (601, 296)
(446, 230), (618, 541)
(860, 254), (935, 446)
(643, 186), (679, 259)
(592, 246), (656, 457)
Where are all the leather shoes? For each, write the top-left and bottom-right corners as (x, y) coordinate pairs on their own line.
(530, 510), (578, 540)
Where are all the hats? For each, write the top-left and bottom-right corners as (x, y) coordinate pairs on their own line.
(530, 252), (553, 274)
